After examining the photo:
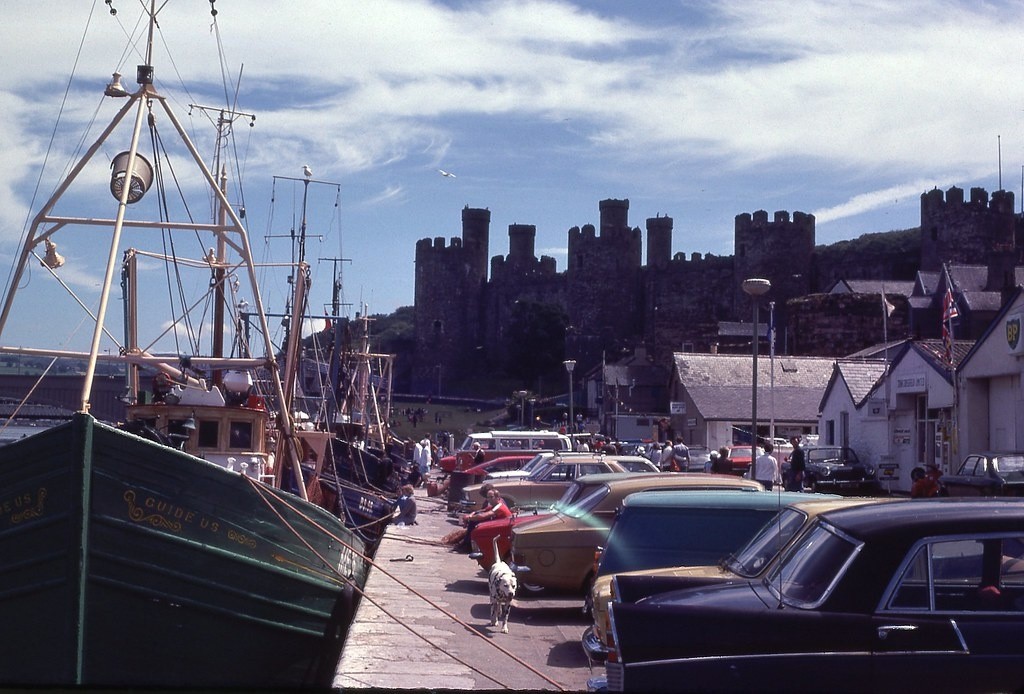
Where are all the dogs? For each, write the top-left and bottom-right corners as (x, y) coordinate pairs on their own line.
(488, 533), (517, 634)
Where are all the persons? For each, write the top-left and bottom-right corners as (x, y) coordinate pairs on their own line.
(409, 433), (451, 477)
(458, 483), (508, 545)
(462, 489), (512, 559)
(750, 443), (778, 491)
(392, 484), (418, 526)
(489, 436), (691, 472)
(784, 436), (805, 493)
(469, 442), (485, 466)
(704, 450), (721, 473)
(558, 413), (590, 435)
(910, 467), (938, 497)
(390, 395), (481, 428)
(711, 447), (732, 474)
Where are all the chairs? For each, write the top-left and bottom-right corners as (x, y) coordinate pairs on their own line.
(978, 586), (1002, 610)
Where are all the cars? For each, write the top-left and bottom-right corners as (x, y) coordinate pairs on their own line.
(727, 445), (765, 476)
(586, 496), (1024, 694)
(780, 445), (876, 497)
(934, 449), (1024, 499)
(581, 498), (1024, 664)
(439, 431), (843, 596)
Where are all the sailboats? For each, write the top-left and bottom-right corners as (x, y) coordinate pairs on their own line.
(0, 0), (411, 693)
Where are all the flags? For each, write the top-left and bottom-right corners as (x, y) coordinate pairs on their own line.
(942, 288), (960, 366)
(765, 308), (778, 357)
(884, 298), (895, 317)
(321, 310), (331, 335)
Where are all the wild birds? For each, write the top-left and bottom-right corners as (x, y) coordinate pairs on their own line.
(302, 165), (312, 180)
(439, 170), (456, 178)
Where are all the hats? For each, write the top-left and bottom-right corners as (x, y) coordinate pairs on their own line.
(711, 450), (718, 457)
(403, 484), (414, 494)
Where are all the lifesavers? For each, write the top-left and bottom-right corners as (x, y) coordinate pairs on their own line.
(118, 425), (178, 450)
(275, 412), (295, 437)
(152, 372), (171, 388)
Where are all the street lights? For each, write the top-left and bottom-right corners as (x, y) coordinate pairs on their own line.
(741, 278), (772, 483)
(528, 399), (536, 431)
(518, 390), (527, 431)
(563, 361), (577, 451)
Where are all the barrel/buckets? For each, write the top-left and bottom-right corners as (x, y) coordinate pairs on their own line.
(265, 410), (316, 430)
(331, 411), (351, 423)
(222, 369), (254, 392)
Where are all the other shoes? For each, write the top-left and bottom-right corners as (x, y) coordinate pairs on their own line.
(457, 544), (471, 553)
